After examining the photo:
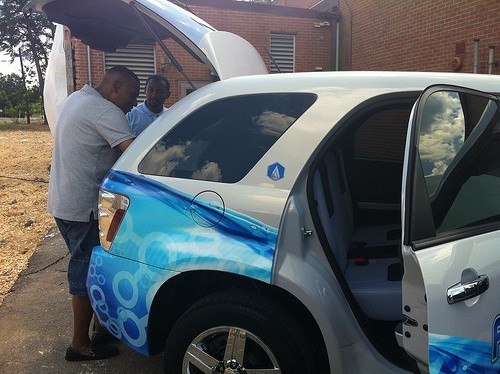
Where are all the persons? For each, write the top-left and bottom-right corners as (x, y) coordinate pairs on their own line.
(44, 62), (143, 364)
(123, 74), (171, 140)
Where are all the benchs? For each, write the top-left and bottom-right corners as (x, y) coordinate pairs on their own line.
(309, 146), (409, 322)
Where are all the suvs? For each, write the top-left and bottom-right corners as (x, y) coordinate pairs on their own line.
(16, 2), (500, 374)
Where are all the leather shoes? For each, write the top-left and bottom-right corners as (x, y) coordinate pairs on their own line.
(92, 333), (119, 343)
(65, 344), (119, 361)
